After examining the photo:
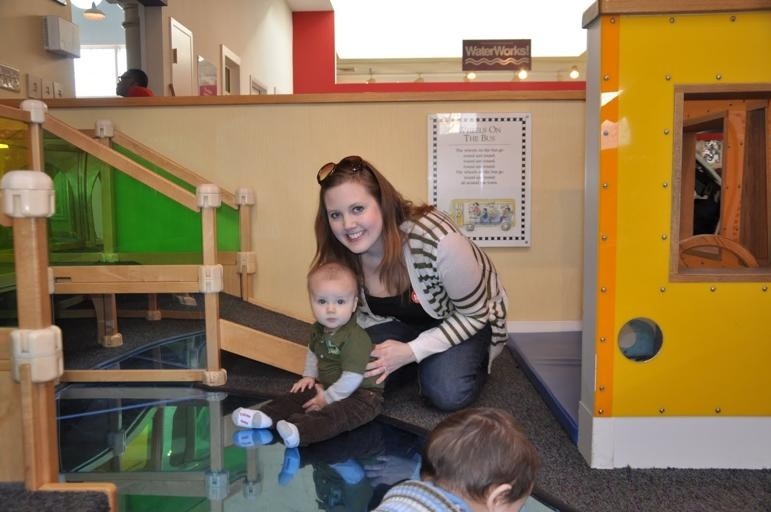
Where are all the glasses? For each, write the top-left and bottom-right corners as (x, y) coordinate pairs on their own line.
(317, 156), (366, 182)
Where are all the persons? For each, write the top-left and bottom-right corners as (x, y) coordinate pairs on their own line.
(116, 69), (157, 97)
(231, 262), (386, 448)
(309, 155), (510, 413)
(368, 406), (542, 511)
